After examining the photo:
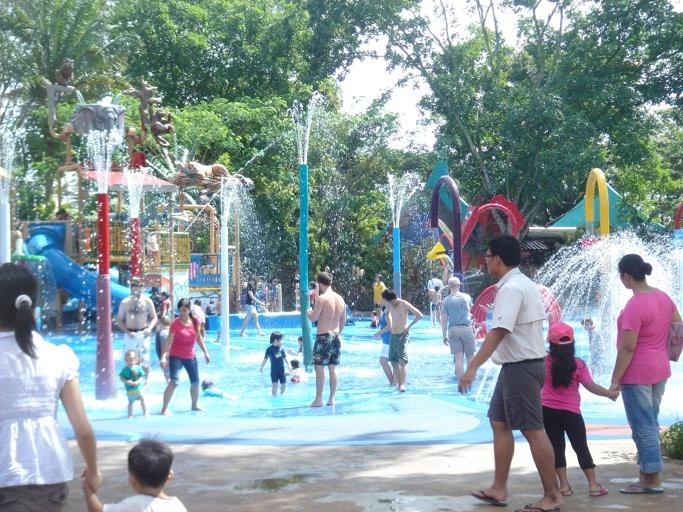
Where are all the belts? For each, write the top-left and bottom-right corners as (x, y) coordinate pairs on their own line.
(126, 328), (147, 331)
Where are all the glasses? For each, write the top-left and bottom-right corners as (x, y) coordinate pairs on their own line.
(484, 254), (498, 258)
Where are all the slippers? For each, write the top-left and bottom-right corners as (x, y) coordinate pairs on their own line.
(470, 483), (664, 512)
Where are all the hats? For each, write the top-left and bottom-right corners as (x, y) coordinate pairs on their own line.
(447, 277), (464, 287)
(547, 323), (574, 345)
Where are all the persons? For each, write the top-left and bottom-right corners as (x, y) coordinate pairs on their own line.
(373, 274), (424, 391)
(307, 272), (346, 406)
(0, 263), (101, 512)
(81, 440), (189, 512)
(240, 276), (303, 397)
(428, 271), (476, 376)
(540, 321), (620, 496)
(459, 234), (566, 512)
(609, 254), (683, 494)
(117, 281), (222, 418)
(581, 316), (607, 383)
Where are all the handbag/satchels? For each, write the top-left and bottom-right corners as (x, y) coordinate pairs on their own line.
(666, 322), (683, 361)
